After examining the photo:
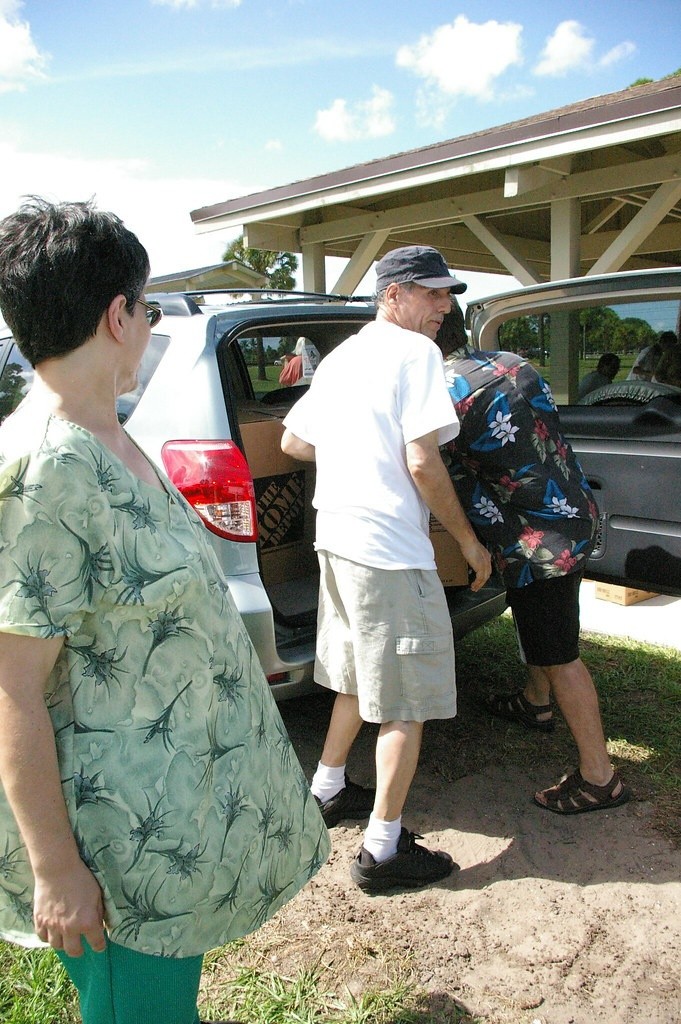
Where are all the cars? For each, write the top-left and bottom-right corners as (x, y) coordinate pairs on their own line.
(519, 347), (550, 361)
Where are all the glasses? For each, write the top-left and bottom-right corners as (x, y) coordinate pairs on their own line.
(135, 298), (163, 328)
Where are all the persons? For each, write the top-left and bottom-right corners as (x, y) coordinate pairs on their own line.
(279, 245), (681, 894)
(0, 194), (332, 1024)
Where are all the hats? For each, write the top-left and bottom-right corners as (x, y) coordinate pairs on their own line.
(374, 243), (468, 295)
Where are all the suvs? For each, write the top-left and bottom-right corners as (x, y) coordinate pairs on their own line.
(0, 266), (681, 701)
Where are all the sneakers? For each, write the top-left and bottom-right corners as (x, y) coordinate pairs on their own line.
(349, 826), (454, 893)
(312, 772), (376, 829)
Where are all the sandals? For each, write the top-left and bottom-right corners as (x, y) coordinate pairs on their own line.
(533, 766), (631, 816)
(485, 691), (556, 734)
